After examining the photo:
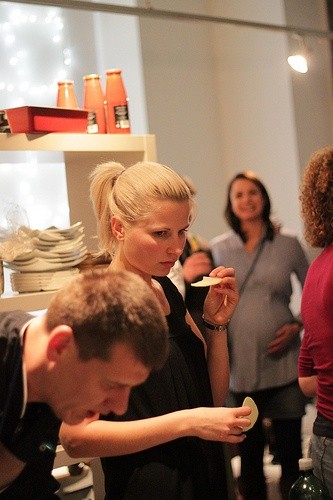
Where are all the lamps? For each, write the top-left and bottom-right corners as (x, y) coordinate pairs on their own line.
(286, 33), (316, 74)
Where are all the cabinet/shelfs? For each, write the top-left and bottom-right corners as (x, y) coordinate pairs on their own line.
(0, 131), (187, 500)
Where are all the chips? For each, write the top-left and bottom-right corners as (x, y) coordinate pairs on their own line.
(240, 397), (259, 432)
(190, 276), (223, 287)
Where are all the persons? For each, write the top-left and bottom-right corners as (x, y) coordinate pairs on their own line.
(58, 161), (253, 500)
(299, 147), (333, 500)
(0, 268), (168, 500)
(205, 170), (310, 500)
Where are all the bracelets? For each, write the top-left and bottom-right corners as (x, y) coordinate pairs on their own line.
(202, 314), (228, 331)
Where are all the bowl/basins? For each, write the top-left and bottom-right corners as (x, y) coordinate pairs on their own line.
(0, 106), (93, 133)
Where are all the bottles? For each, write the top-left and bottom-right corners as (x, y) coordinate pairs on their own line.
(104, 70), (130, 134)
(57, 81), (78, 109)
(83, 74), (107, 134)
(289, 458), (327, 500)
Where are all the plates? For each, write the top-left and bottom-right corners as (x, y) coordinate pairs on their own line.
(1, 222), (88, 292)
